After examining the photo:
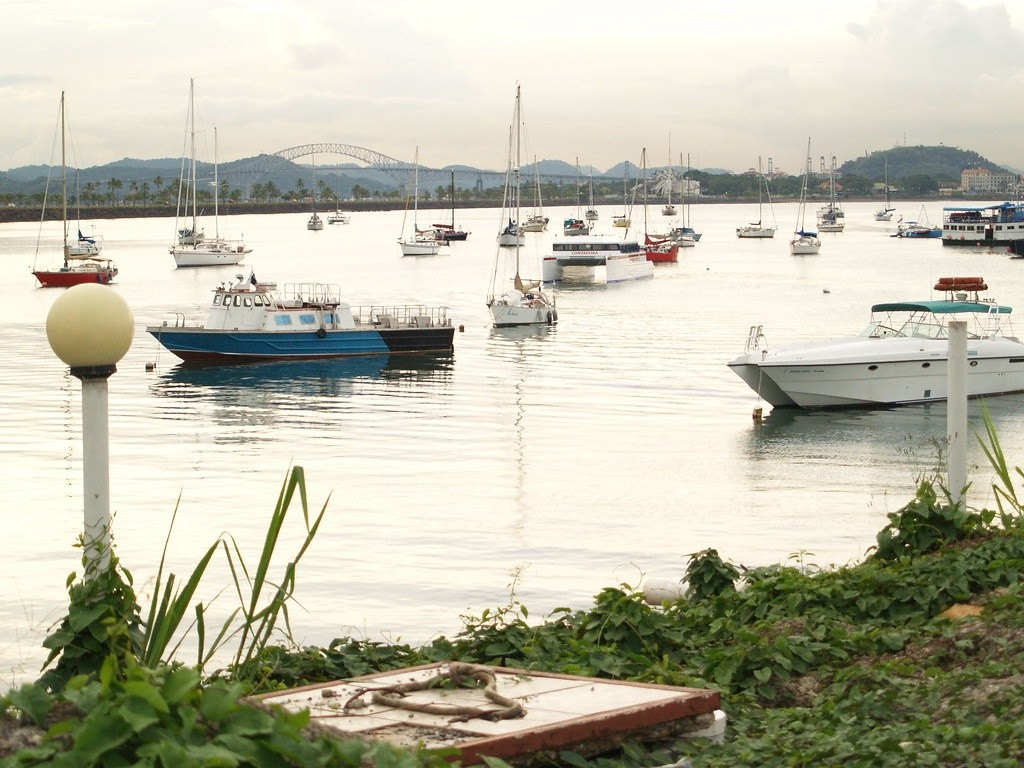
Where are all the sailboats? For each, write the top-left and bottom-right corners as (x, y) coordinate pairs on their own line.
(179, 74), (205, 247)
(789, 136), (822, 255)
(27, 92), (119, 288)
(561, 156), (593, 238)
(394, 146), (470, 255)
(68, 169), (102, 258)
(306, 153), (325, 231)
(814, 156), (848, 234)
(871, 156), (895, 222)
(583, 170), (599, 220)
(637, 129), (702, 263)
(484, 80), (559, 329)
(735, 153), (779, 238)
(327, 170), (351, 224)
(612, 176), (632, 230)
(168, 126), (253, 267)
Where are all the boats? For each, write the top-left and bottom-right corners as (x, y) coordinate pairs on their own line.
(727, 275), (1024, 410)
(936, 199), (1024, 246)
(143, 265), (455, 373)
(539, 233), (654, 286)
(1007, 239), (1024, 258)
(889, 204), (942, 240)
